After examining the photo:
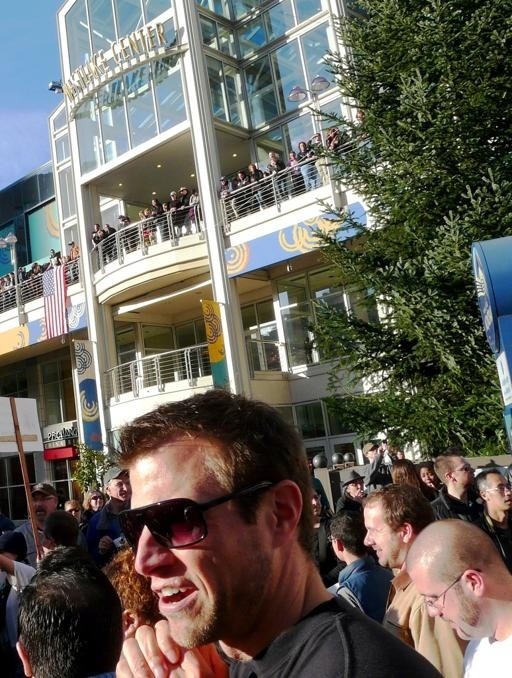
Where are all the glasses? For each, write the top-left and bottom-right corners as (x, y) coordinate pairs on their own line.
(449, 463), (472, 474)
(90, 495), (103, 500)
(483, 483), (510, 494)
(32, 497), (54, 504)
(115, 479), (278, 552)
(417, 567), (483, 606)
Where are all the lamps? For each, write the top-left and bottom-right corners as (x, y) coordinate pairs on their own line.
(0, 233), (27, 324)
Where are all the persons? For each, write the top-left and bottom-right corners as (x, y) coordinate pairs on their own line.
(91, 186), (199, 264)
(0, 241), (79, 313)
(220, 111), (375, 220)
(107, 390), (440, 676)
(312, 440), (512, 677)
(1, 468), (232, 678)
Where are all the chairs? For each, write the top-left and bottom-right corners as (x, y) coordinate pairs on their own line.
(288, 74), (340, 210)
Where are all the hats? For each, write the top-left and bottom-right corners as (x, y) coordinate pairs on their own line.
(30, 481), (58, 496)
(0, 532), (27, 555)
(341, 470), (366, 487)
(362, 443), (379, 455)
(103, 467), (130, 483)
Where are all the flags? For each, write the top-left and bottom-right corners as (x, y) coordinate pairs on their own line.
(42, 263), (68, 339)
